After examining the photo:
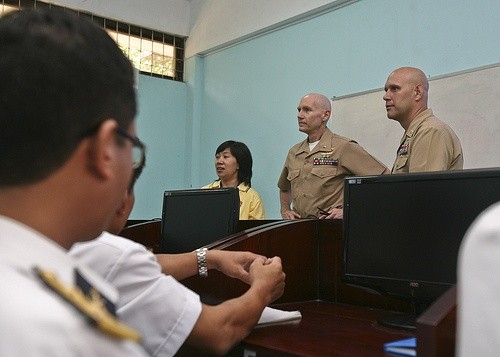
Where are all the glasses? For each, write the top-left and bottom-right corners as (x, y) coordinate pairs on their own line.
(89, 122), (146, 180)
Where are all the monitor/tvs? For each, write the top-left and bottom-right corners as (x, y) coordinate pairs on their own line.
(342, 167), (500, 332)
(158, 188), (241, 255)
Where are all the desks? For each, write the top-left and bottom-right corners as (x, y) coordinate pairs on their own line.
(119, 216), (459, 357)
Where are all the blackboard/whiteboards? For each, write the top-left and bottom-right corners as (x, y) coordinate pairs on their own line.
(325, 62), (499, 169)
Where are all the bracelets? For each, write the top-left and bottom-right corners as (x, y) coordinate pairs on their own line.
(195, 247), (208, 277)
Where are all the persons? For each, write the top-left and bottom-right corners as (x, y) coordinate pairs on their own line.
(0, 5), (151, 357)
(201, 140), (264, 220)
(67, 154), (286, 357)
(277, 93), (390, 220)
(382, 67), (464, 175)
